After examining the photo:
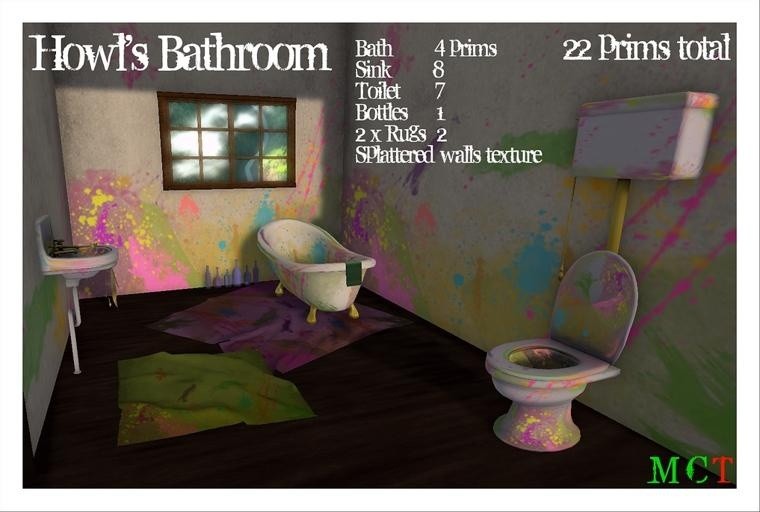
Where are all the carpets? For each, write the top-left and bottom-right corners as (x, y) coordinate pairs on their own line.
(143, 279), (414, 372)
(115, 352), (317, 447)
